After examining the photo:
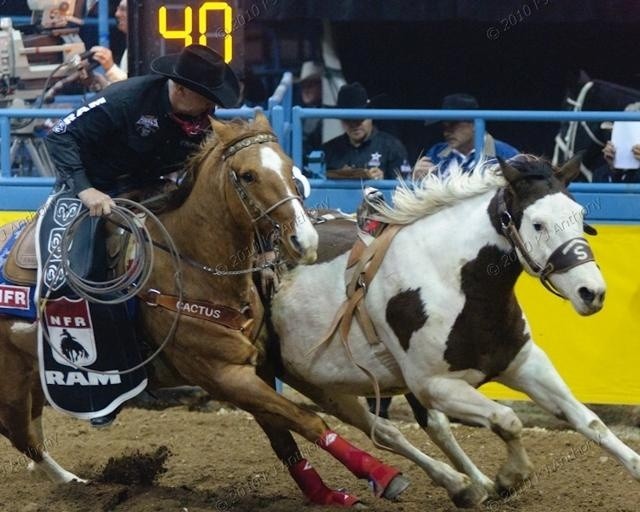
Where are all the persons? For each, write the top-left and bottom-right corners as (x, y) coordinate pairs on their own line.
(407, 90), (523, 182)
(318, 82), (411, 180)
(601, 101), (640, 183)
(35, 43), (240, 428)
(291, 60), (323, 166)
(91, 0), (127, 84)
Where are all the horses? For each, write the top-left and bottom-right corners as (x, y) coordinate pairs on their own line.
(0, 110), (412, 509)
(266, 146), (640, 510)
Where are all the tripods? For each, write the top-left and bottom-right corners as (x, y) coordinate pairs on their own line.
(10, 59), (101, 177)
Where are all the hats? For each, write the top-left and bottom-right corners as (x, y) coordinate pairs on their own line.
(293, 61), (322, 84)
(152, 44), (240, 108)
(320, 82), (385, 108)
(424, 94), (478, 127)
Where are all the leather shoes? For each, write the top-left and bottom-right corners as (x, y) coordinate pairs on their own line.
(90, 403), (122, 427)
